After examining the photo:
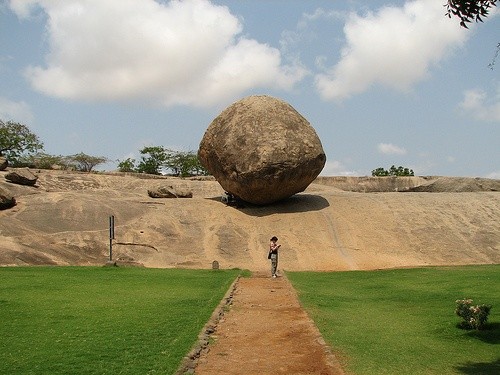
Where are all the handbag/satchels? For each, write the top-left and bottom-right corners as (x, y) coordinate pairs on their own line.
(268, 252), (272, 260)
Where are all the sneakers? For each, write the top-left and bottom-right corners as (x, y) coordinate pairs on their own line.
(272, 274), (277, 278)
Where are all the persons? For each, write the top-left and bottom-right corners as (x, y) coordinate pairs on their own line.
(268, 237), (281, 278)
